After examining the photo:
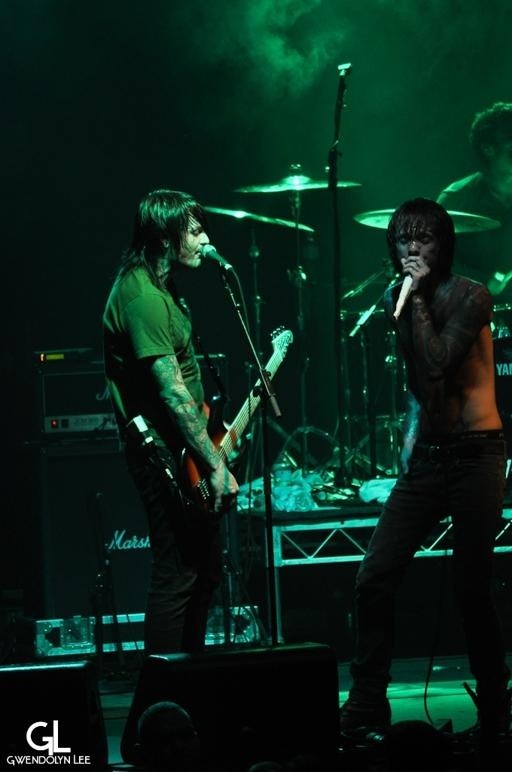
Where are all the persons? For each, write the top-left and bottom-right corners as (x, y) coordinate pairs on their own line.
(101, 188), (242, 769)
(435, 102), (511, 303)
(337, 195), (509, 735)
(380, 719), (442, 772)
(135, 699), (206, 770)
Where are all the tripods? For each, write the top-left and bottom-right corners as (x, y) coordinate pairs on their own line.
(241, 202), (409, 481)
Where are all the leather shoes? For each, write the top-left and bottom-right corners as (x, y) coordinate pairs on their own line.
(337, 698), (392, 730)
(464, 688), (512, 743)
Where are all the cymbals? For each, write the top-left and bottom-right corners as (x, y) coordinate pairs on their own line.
(353, 206), (499, 234)
(234, 174), (362, 194)
(202, 205), (314, 232)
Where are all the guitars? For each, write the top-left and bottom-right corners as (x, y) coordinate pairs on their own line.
(176, 325), (294, 518)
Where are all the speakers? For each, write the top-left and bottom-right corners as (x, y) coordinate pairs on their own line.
(0, 661), (108, 772)
(21, 439), (230, 646)
(120, 640), (340, 770)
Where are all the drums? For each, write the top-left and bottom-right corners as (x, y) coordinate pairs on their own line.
(338, 307), (408, 416)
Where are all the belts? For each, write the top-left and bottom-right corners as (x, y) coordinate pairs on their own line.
(417, 429), (505, 446)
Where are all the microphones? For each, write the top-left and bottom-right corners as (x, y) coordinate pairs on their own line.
(392, 273), (414, 320)
(201, 244), (231, 270)
